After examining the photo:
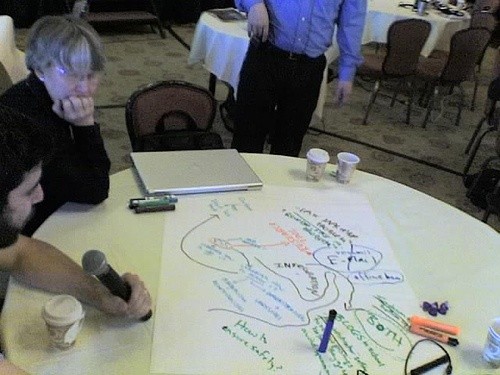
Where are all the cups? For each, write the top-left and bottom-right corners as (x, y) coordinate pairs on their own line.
(337, 151), (360, 184)
(482, 318), (500, 368)
(417, 0), (426, 16)
(41, 294), (82, 349)
(457, 0), (467, 10)
(306, 148), (330, 181)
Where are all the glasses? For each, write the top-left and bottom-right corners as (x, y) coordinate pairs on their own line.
(46, 62), (105, 83)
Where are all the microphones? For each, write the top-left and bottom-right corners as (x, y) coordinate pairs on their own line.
(82, 250), (152, 322)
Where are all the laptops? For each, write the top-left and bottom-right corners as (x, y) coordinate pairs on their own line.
(129, 149), (263, 195)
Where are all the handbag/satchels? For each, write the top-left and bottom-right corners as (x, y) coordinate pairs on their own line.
(135, 109), (224, 151)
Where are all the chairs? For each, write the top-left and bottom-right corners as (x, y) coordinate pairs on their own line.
(125, 79), (217, 152)
(359, 12), (500, 223)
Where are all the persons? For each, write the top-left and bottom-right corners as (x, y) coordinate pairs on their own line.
(0, 102), (152, 375)
(0, 13), (110, 238)
(230, 0), (367, 161)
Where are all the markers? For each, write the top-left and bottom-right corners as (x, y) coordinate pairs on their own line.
(319, 308), (337, 354)
(410, 316), (460, 335)
(408, 326), (458, 347)
(129, 194), (178, 213)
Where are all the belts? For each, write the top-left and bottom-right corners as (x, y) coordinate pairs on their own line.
(262, 39), (325, 61)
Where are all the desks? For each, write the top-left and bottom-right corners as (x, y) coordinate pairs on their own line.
(0, 152), (500, 375)
(361, 0), (472, 57)
(189, 10), (340, 125)
